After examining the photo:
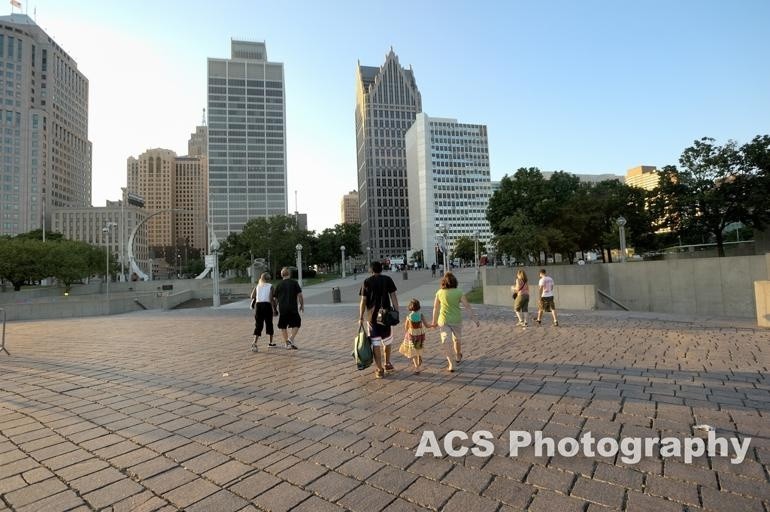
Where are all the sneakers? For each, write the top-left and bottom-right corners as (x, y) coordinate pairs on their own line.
(532, 318), (541, 324)
(551, 320), (558, 327)
(515, 320), (528, 327)
(375, 368), (385, 380)
(383, 362), (395, 373)
(251, 342), (259, 353)
(448, 365), (455, 372)
(268, 343), (277, 348)
(456, 351), (463, 364)
(285, 339), (299, 351)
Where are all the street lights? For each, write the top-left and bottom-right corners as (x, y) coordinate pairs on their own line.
(101, 226), (109, 297)
(340, 246), (346, 278)
(210, 240), (220, 307)
(472, 231), (478, 278)
(295, 245), (303, 289)
(616, 217), (627, 262)
(366, 247), (370, 265)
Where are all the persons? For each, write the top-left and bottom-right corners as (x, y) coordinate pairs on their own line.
(249, 271), (275, 352)
(431, 262), (436, 278)
(274, 268), (304, 349)
(353, 266), (358, 280)
(514, 269), (531, 328)
(438, 263), (444, 276)
(535, 269), (559, 327)
(380, 254), (421, 273)
(406, 299), (434, 376)
(431, 273), (479, 373)
(360, 261), (399, 380)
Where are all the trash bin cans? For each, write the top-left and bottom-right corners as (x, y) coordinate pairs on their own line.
(439, 270), (443, 276)
(402, 270), (408, 280)
(332, 287), (341, 303)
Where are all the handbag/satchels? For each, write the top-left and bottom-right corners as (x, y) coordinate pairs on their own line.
(250, 298), (256, 310)
(376, 307), (401, 327)
(350, 322), (374, 372)
(512, 292), (518, 300)
